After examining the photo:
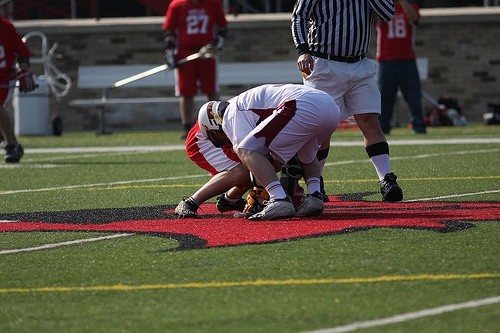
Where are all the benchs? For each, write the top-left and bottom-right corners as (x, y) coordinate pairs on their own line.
(67, 57), (427, 136)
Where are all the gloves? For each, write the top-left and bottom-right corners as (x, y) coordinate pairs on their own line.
(163, 42), (177, 70)
(198, 43), (217, 60)
(15, 69), (36, 93)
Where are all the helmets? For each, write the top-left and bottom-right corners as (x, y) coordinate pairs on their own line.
(198, 100), (232, 148)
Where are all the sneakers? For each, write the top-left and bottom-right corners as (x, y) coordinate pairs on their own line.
(215, 194), (247, 213)
(174, 196), (203, 219)
(247, 195), (295, 220)
(295, 191), (324, 217)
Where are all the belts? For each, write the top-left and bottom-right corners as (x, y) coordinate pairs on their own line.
(309, 51), (366, 63)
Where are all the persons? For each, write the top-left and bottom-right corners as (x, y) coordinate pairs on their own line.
(162, 0), (229, 143)
(173, 83), (340, 220)
(291, 0), (403, 203)
(373, 0), (429, 134)
(0, 15), (36, 163)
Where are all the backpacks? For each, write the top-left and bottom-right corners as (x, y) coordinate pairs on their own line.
(429, 96), (462, 126)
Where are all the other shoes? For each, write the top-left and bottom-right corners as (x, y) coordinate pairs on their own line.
(180, 127), (194, 140)
(319, 175), (329, 202)
(412, 124), (427, 134)
(3, 144), (24, 165)
(378, 172), (403, 202)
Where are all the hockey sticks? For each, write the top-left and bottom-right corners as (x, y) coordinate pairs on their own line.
(0, 83), (40, 90)
(420, 90), (467, 127)
(113, 44), (218, 89)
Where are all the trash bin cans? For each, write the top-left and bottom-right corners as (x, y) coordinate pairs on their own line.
(14, 79), (51, 136)
